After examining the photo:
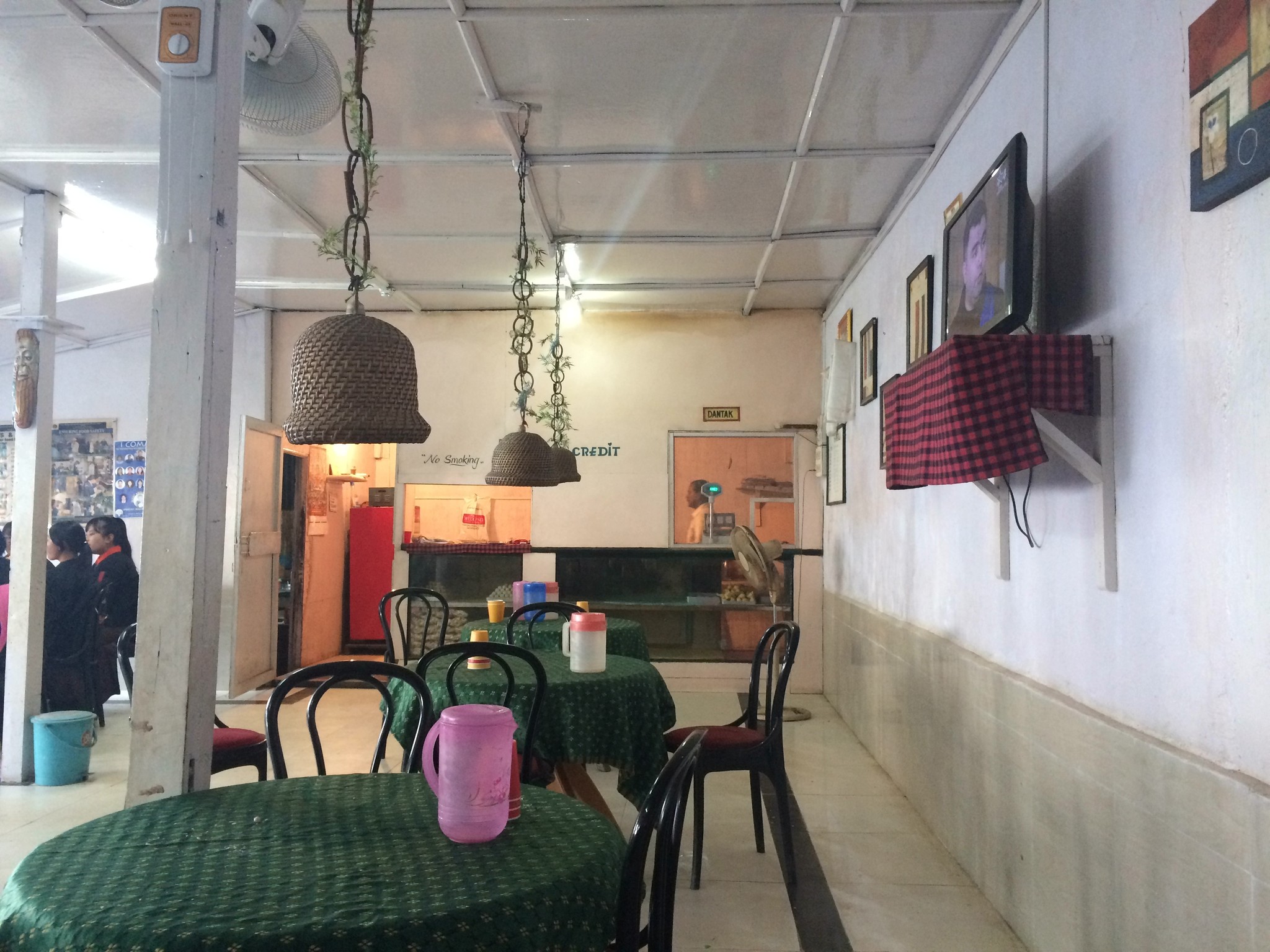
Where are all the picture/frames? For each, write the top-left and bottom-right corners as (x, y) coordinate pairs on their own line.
(906, 254), (934, 370)
(879, 373), (900, 470)
(825, 423), (847, 506)
(836, 308), (851, 342)
(859, 318), (878, 406)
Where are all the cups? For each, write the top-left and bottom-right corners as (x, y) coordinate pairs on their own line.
(576, 601), (590, 613)
(466, 630), (492, 670)
(506, 738), (520, 821)
(487, 599), (506, 624)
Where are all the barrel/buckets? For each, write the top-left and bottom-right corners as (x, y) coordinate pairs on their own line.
(30, 711), (97, 786)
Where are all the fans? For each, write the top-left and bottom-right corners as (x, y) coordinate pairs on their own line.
(727, 523), (814, 723)
(239, 1), (344, 136)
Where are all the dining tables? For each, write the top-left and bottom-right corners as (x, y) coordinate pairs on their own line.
(0, 772), (634, 952)
(381, 647), (678, 905)
(462, 614), (651, 665)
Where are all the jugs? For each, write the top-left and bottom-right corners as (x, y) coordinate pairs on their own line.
(421, 703), (520, 845)
(513, 579), (559, 622)
(561, 611), (607, 673)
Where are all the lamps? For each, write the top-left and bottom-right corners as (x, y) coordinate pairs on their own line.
(481, 100), (561, 487)
(281, 0), (437, 447)
(548, 233), (583, 483)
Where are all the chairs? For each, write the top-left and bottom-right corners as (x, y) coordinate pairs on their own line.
(265, 659), (436, 779)
(506, 601), (587, 651)
(377, 587), (450, 773)
(664, 618), (801, 892)
(415, 641), (546, 783)
(613, 726), (714, 952)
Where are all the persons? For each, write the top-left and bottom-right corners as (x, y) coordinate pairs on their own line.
(90, 516), (139, 662)
(41, 521), (93, 715)
(686, 480), (715, 543)
(0, 529), (9, 727)
(51, 433), (147, 520)
(940, 200), (1005, 341)
(2, 521), (55, 589)
